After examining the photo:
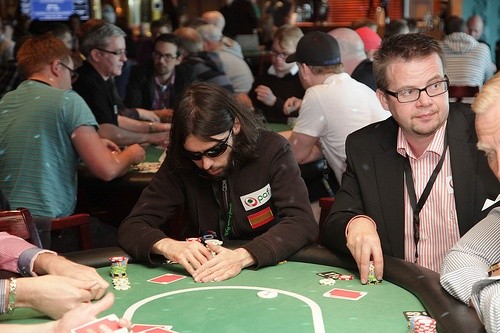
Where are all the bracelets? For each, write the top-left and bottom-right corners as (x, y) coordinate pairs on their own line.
(7, 277), (16, 315)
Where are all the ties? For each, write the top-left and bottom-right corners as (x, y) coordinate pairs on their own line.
(155, 82), (172, 109)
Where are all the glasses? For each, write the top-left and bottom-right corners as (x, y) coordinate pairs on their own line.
(382, 75), (450, 103)
(49, 59), (78, 84)
(181, 117), (233, 161)
(266, 46), (289, 59)
(89, 45), (128, 57)
(152, 50), (179, 63)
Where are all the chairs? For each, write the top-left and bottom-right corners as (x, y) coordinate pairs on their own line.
(0, 207), (113, 253)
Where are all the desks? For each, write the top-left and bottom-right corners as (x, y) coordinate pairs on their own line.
(244, 51), (272, 77)
(76, 120), (328, 228)
(0, 239), (488, 333)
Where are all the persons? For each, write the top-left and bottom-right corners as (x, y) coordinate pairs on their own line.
(286, 32), (393, 186)
(359, 14), (491, 58)
(116, 82), (319, 283)
(173, 27), (235, 93)
(0, 35), (146, 250)
(0, 231), (109, 319)
(72, 24), (171, 144)
(441, 15), (497, 97)
(235, 25), (306, 124)
(283, 28), (376, 197)
(0, 13), (207, 91)
(0, 292), (133, 333)
(200, 11), (244, 59)
(127, 34), (197, 124)
(197, 25), (254, 92)
(320, 35), (500, 284)
(218, 0), (329, 40)
(437, 74), (500, 333)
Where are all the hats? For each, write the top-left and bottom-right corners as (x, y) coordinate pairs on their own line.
(355, 26), (383, 51)
(285, 30), (341, 66)
(196, 24), (222, 41)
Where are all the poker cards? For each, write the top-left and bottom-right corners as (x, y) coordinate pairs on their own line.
(323, 288), (367, 301)
(147, 273), (187, 284)
(71, 314), (178, 333)
